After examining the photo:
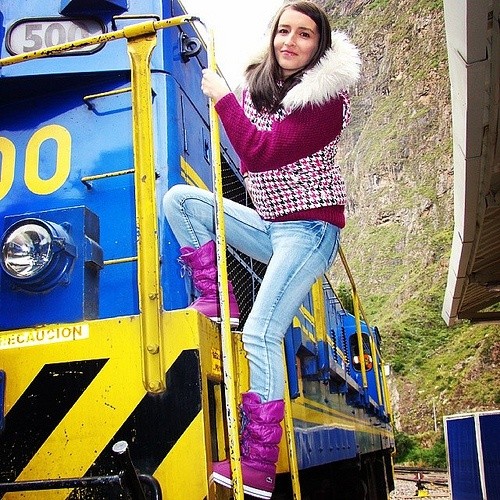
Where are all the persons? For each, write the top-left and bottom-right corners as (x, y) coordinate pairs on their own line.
(163, 2), (361, 500)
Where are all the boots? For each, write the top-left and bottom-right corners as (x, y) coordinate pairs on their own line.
(181, 240), (240, 327)
(212, 392), (285, 500)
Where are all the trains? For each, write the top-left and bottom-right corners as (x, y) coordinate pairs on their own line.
(0, 0), (397, 500)
